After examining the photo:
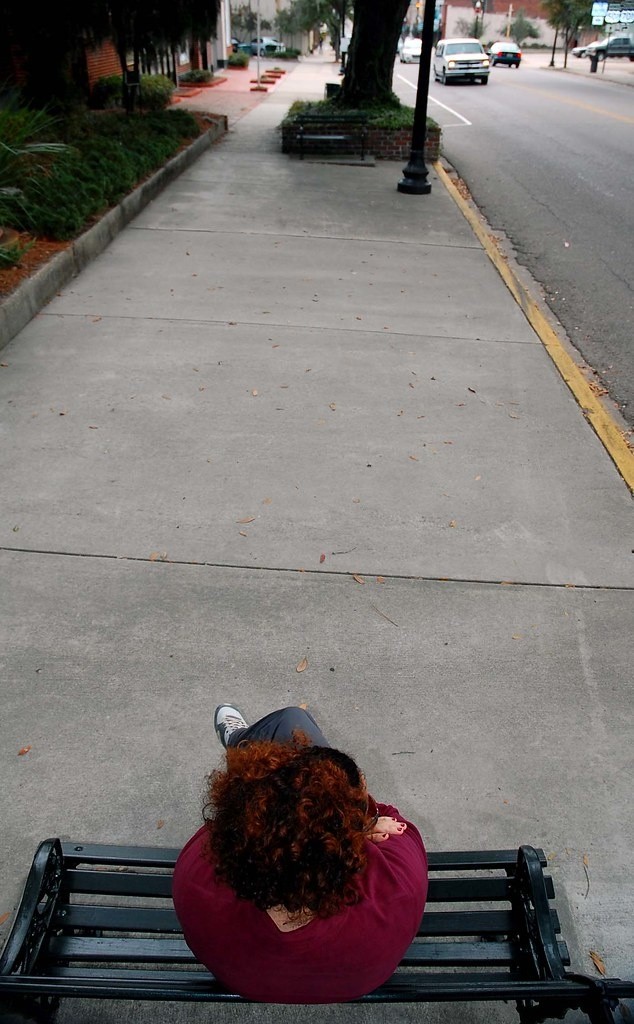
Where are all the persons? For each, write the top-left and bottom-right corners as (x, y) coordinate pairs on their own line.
(172, 703), (427, 1004)
(319, 35), (323, 48)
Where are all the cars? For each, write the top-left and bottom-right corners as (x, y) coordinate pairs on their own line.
(433, 38), (491, 85)
(238, 37), (286, 57)
(488, 42), (522, 68)
(585, 37), (634, 62)
(399, 41), (422, 64)
(231, 37), (244, 53)
(572, 41), (602, 59)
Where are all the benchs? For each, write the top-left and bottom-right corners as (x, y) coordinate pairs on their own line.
(0, 838), (634, 1024)
(295, 114), (369, 161)
